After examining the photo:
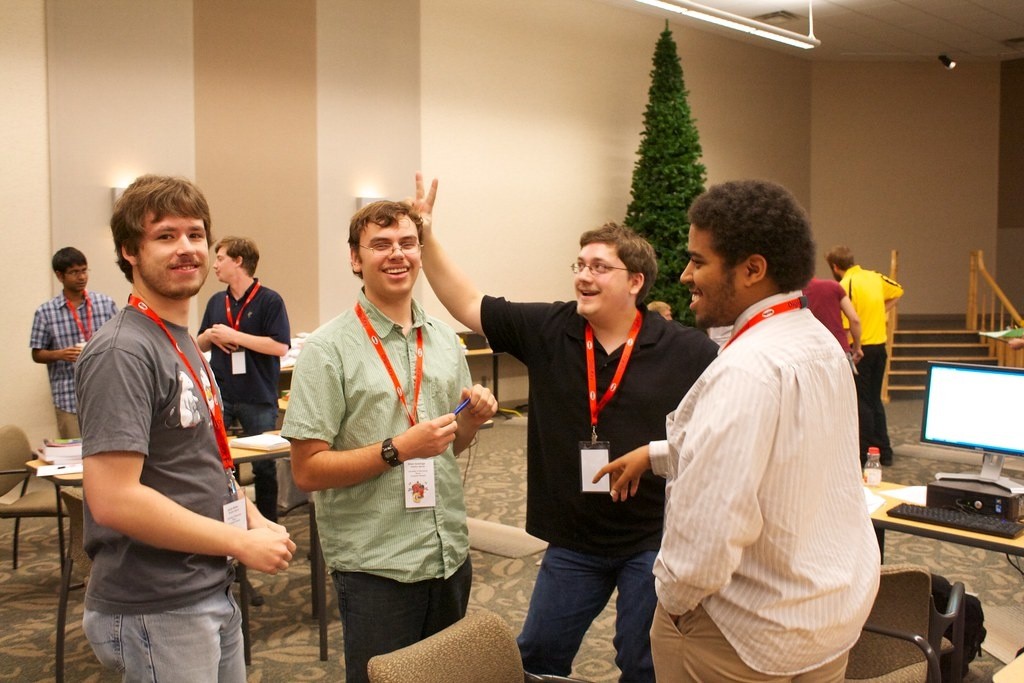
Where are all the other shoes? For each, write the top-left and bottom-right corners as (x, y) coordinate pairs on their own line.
(881, 458), (892, 466)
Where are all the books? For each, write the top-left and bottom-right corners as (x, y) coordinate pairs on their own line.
(24, 435), (84, 475)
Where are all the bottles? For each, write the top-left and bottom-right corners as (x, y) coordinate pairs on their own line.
(863, 447), (883, 485)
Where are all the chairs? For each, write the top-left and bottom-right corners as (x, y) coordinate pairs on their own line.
(0, 427), (86, 588)
(368, 609), (594, 683)
(55, 489), (251, 683)
(844, 563), (965, 683)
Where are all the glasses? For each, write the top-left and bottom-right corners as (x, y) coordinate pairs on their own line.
(62, 268), (91, 277)
(358, 242), (423, 257)
(571, 258), (633, 276)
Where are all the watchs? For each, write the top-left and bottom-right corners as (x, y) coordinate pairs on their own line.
(381, 437), (402, 468)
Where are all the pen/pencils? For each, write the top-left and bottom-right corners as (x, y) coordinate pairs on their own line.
(453, 399), (471, 415)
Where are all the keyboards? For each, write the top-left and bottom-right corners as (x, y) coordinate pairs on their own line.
(886, 502), (1024, 539)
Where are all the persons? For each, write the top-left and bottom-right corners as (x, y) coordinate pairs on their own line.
(281, 200), (497, 683)
(1008, 338), (1024, 351)
(402, 173), (720, 683)
(28, 247), (117, 438)
(824, 247), (904, 469)
(803, 275), (864, 374)
(648, 178), (881, 683)
(194, 236), (291, 525)
(73, 173), (296, 683)
(646, 301), (673, 321)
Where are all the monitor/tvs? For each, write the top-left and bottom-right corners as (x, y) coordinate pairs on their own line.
(919, 360), (1024, 493)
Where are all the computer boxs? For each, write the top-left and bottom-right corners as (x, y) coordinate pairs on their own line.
(925, 479), (1024, 522)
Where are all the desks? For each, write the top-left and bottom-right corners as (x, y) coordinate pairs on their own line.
(862, 480), (1024, 566)
(26, 431), (327, 619)
(457, 337), (511, 419)
(201, 336), (310, 432)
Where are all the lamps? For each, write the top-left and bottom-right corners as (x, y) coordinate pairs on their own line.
(635, 0), (821, 50)
(938, 55), (957, 69)
